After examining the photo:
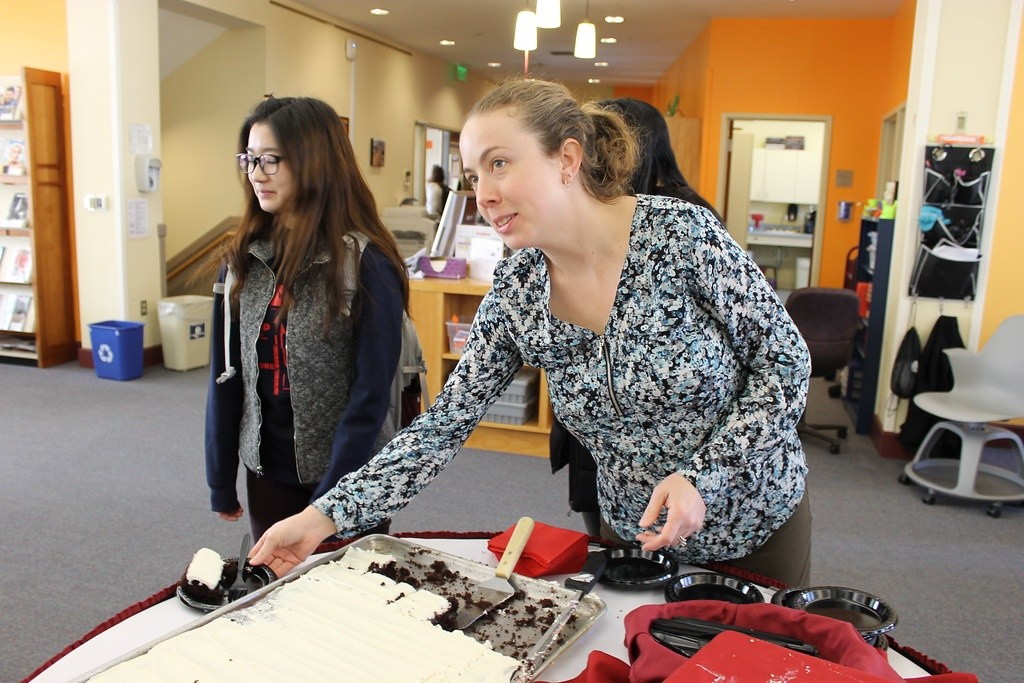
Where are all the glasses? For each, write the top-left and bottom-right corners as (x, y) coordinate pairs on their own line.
(236, 153), (285, 175)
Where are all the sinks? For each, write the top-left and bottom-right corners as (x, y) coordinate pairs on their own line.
(764, 230), (796, 235)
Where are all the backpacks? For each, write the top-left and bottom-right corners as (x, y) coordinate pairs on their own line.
(335, 231), (430, 454)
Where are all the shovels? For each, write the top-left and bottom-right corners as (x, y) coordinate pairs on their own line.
(452, 516), (534, 629)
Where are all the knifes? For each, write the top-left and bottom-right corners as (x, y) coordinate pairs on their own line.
(526, 551), (606, 660)
(650, 618), (819, 658)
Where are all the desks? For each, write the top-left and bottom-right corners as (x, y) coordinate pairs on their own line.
(19, 531), (981, 683)
(748, 232), (813, 252)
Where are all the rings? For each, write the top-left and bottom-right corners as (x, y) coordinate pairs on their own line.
(678, 536), (686, 542)
(680, 542), (687, 547)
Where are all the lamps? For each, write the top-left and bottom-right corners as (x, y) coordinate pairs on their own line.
(513, 0), (597, 60)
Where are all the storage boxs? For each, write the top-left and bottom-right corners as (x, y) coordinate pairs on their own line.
(445, 320), (540, 425)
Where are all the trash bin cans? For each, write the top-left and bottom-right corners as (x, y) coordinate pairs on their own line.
(88, 320), (145, 380)
(158, 295), (215, 371)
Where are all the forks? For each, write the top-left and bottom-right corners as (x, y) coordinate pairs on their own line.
(227, 534), (251, 604)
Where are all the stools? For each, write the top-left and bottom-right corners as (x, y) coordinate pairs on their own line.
(756, 248), (783, 291)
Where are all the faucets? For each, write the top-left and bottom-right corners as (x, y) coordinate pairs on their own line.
(780, 213), (789, 229)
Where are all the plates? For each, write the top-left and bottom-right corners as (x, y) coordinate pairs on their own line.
(665, 572), (765, 603)
(771, 586), (899, 647)
(593, 545), (678, 590)
(176, 558), (276, 613)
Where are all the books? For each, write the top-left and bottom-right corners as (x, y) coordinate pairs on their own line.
(0, 85), (32, 330)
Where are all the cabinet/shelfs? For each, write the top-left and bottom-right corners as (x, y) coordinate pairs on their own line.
(0, 65), (83, 372)
(407, 276), (555, 457)
(845, 215), (893, 437)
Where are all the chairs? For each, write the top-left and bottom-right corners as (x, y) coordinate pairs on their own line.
(900, 313), (1024, 518)
(783, 287), (860, 456)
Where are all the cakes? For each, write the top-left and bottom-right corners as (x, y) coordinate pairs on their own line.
(181, 548), (227, 605)
(77, 545), (524, 683)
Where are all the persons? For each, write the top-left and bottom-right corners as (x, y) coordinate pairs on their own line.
(204, 97), (413, 543)
(549, 96), (721, 537)
(427, 163), (452, 214)
(1, 144), (25, 176)
(247, 80), (813, 593)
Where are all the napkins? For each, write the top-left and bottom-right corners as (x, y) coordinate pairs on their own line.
(488, 517), (588, 578)
